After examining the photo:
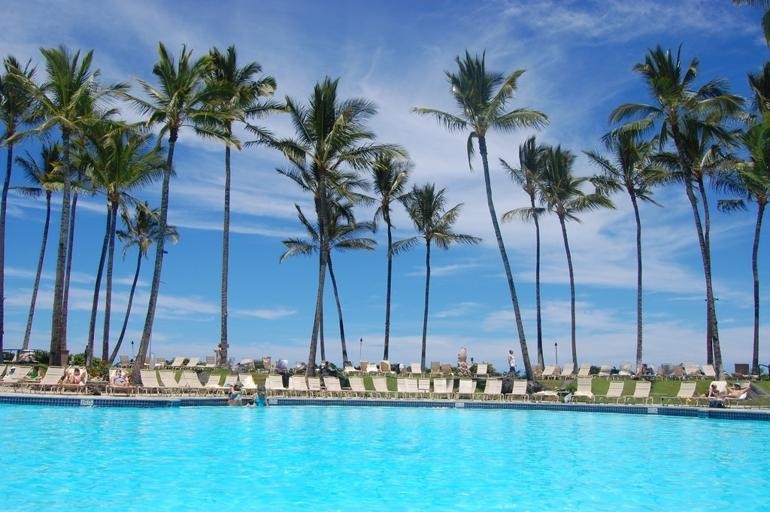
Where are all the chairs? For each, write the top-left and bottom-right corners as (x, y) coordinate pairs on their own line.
(0, 347), (770, 408)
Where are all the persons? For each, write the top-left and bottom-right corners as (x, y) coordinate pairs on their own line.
(64, 367), (82, 383)
(632, 362), (650, 380)
(712, 384), (751, 399)
(698, 383), (717, 400)
(229, 383), (242, 407)
(214, 344), (222, 365)
(231, 354), (341, 374)
(251, 384), (270, 407)
(457, 361), (469, 377)
(111, 368), (130, 387)
(507, 349), (515, 372)
